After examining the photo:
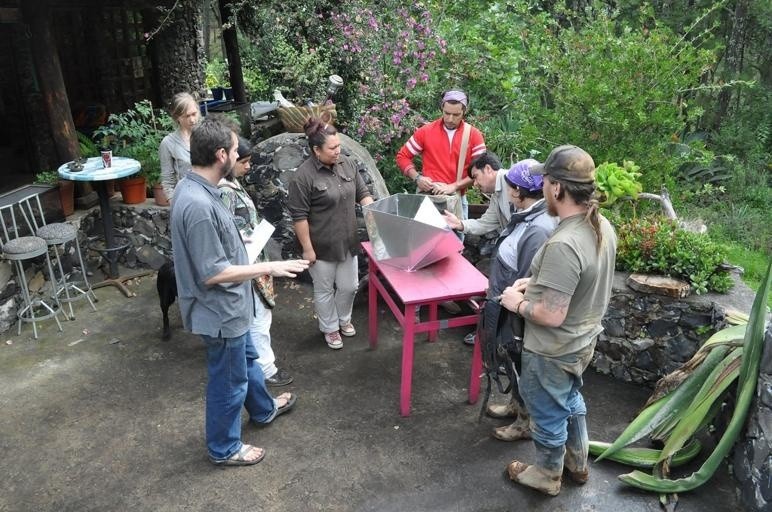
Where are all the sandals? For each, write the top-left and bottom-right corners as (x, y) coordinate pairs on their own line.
(275, 392), (297, 415)
(209, 445), (265, 466)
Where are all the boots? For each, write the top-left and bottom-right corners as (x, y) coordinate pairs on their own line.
(489, 397), (589, 496)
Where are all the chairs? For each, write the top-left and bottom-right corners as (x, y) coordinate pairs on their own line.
(19, 192), (98, 321)
(0, 202), (69, 339)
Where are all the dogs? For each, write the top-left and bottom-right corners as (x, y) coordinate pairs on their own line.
(157, 258), (178, 342)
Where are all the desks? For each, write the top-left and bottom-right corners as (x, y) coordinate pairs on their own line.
(361, 241), (489, 417)
(57, 156), (152, 298)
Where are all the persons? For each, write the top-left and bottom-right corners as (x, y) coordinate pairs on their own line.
(395, 91), (487, 255)
(507, 144), (617, 497)
(168, 121), (310, 464)
(442, 156), (559, 440)
(285, 118), (375, 349)
(160, 91), (200, 202)
(217, 136), (293, 387)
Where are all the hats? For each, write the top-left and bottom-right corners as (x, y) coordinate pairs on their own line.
(530, 145), (595, 183)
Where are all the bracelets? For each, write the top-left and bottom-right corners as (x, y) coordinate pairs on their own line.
(414, 174), (421, 183)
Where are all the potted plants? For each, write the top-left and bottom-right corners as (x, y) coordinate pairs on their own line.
(36, 170), (74, 217)
(91, 99), (153, 204)
(132, 142), (172, 205)
(223, 79), (234, 100)
(207, 73), (223, 101)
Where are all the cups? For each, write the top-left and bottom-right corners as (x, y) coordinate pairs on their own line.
(100, 148), (113, 168)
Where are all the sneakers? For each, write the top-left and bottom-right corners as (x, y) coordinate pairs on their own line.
(325, 323), (356, 349)
(439, 301), (461, 315)
(265, 370), (293, 386)
(464, 331), (478, 344)
